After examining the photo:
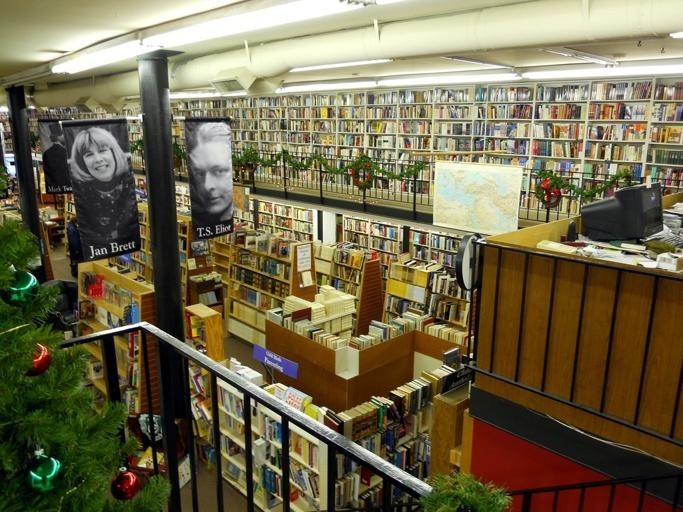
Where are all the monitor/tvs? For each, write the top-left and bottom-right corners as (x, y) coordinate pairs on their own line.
(581, 182), (663, 244)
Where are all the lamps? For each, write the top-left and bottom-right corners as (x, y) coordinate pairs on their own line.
(0, 1), (683, 99)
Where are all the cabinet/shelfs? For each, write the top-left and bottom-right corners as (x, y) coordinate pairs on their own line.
(0, 76), (683, 512)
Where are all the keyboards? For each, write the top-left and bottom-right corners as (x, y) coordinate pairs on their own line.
(664, 236), (683, 248)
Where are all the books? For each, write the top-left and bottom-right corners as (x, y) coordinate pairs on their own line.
(175, 183), (472, 512)
(72, 270), (165, 469)
(1, 101), (155, 285)
(171, 79), (683, 222)
